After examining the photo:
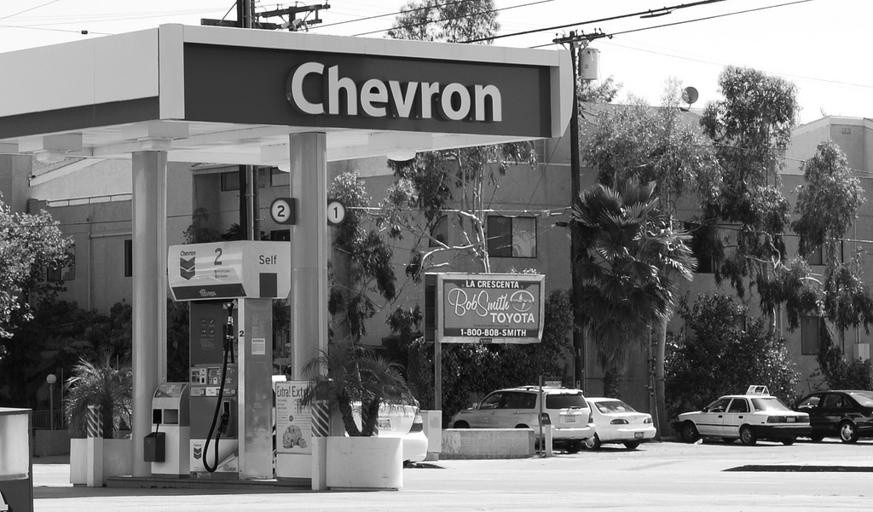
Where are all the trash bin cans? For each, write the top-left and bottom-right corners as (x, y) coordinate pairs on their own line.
(0, 407), (33, 512)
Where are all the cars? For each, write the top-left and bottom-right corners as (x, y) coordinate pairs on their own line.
(674, 385), (814, 446)
(337, 384), (429, 466)
(578, 395), (656, 451)
(790, 389), (872, 444)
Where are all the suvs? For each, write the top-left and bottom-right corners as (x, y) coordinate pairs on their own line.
(448, 385), (596, 454)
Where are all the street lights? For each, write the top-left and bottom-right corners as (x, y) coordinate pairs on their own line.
(44, 373), (56, 430)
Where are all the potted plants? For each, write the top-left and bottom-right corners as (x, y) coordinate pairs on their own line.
(55, 354), (144, 490)
(296, 333), (421, 492)
